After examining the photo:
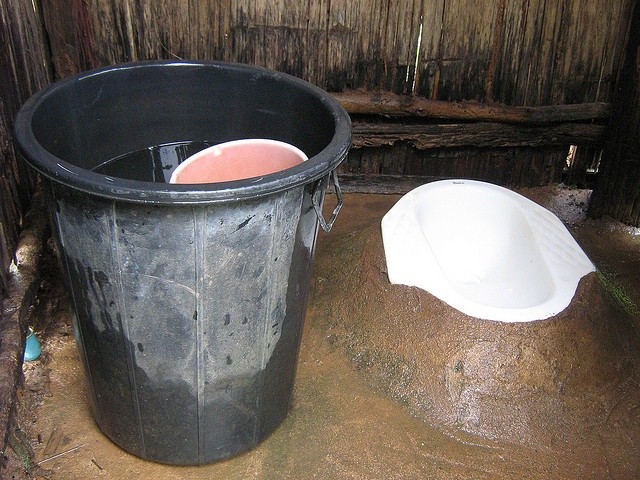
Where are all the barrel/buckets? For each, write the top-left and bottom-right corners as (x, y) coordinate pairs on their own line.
(11, 60), (353, 466)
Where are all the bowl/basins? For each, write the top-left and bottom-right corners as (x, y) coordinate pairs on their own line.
(168, 139), (309, 185)
